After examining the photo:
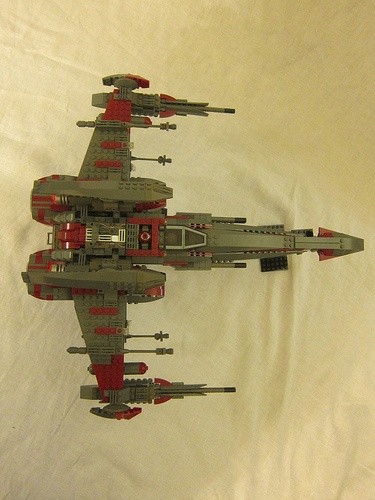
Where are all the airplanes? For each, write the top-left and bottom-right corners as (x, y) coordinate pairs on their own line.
(22, 74), (364, 421)
